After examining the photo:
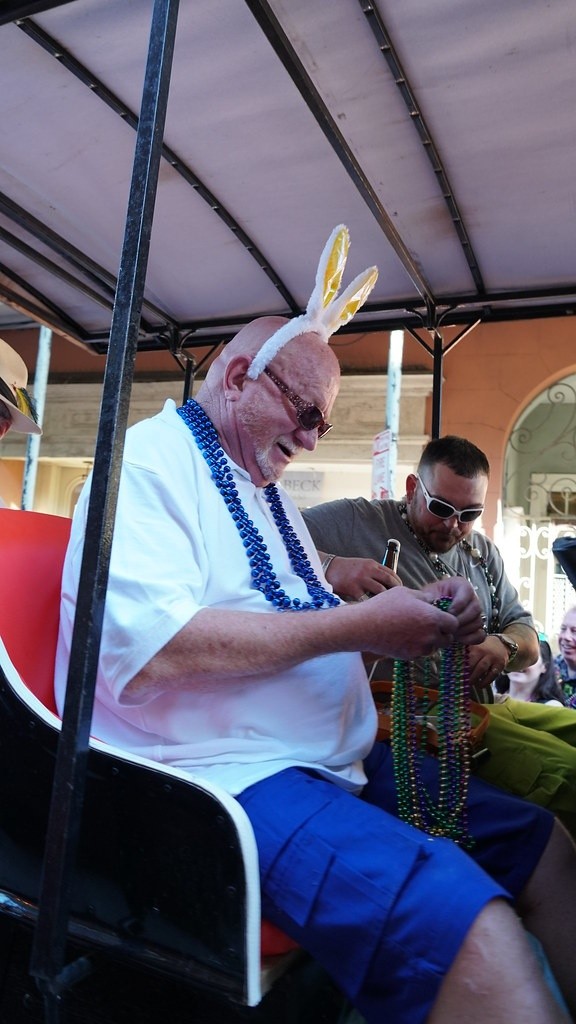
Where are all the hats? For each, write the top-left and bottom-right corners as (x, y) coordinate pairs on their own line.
(0, 338), (42, 435)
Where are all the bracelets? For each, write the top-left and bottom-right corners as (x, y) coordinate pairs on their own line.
(322, 553), (337, 575)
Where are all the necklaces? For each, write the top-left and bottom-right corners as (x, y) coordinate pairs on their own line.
(176, 396), (340, 611)
(390, 595), (476, 850)
(397, 500), (501, 635)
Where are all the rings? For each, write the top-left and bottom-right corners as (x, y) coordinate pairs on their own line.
(492, 667), (499, 675)
(358, 594), (369, 602)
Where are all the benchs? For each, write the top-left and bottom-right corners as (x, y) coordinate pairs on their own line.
(0, 508), (297, 1008)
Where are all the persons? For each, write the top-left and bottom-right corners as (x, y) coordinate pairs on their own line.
(53, 224), (576, 1024)
(0, 336), (44, 444)
(495, 620), (564, 704)
(300, 434), (576, 813)
(551, 606), (576, 707)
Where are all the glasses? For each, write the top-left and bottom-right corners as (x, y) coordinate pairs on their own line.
(416, 473), (484, 523)
(263, 366), (333, 440)
(538, 632), (547, 640)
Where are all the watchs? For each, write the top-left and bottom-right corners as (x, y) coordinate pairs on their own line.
(490, 633), (520, 664)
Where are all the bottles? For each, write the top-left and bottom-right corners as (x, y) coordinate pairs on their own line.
(369, 538), (402, 598)
